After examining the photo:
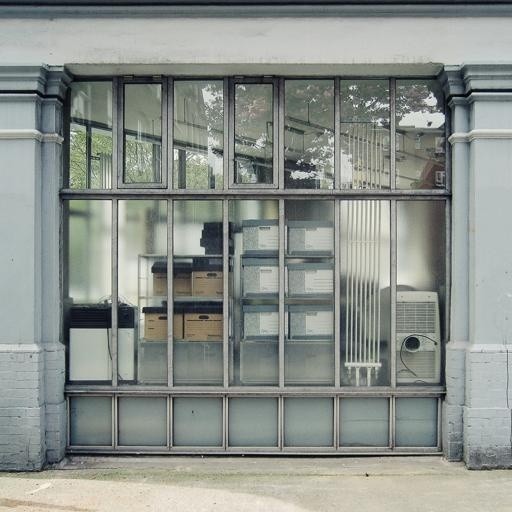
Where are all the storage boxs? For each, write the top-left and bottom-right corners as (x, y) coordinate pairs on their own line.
(137, 218), (335, 384)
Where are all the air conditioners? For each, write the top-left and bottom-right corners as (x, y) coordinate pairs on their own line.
(387, 293), (443, 387)
